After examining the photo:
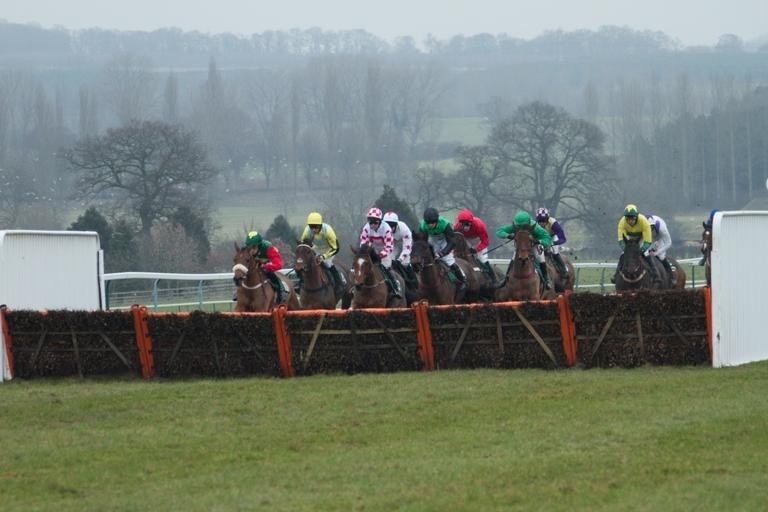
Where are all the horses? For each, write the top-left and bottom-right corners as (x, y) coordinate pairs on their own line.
(233, 240), (300, 313)
(293, 238), (354, 309)
(495, 221), (556, 302)
(614, 233), (669, 293)
(661, 256), (686, 291)
(349, 229), (505, 309)
(545, 248), (574, 294)
(700, 222), (713, 286)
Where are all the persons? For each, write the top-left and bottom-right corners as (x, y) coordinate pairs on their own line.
(610, 203), (720, 287)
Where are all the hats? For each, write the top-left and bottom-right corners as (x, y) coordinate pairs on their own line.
(706, 208), (718, 226)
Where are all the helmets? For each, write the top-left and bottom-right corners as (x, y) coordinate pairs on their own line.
(382, 211), (400, 224)
(366, 207), (382, 221)
(423, 207), (439, 222)
(244, 231), (263, 248)
(623, 203), (639, 217)
(306, 212), (323, 226)
(457, 208), (474, 223)
(535, 207), (550, 222)
(513, 210), (531, 226)
(644, 214), (655, 226)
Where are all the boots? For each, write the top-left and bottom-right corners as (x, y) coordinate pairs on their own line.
(329, 264), (344, 295)
(662, 257), (680, 280)
(610, 254), (626, 283)
(506, 259), (514, 281)
(379, 263), (404, 299)
(268, 272), (288, 304)
(553, 252), (570, 280)
(647, 256), (663, 282)
(450, 262), (470, 293)
(540, 261), (553, 290)
(484, 261), (500, 289)
